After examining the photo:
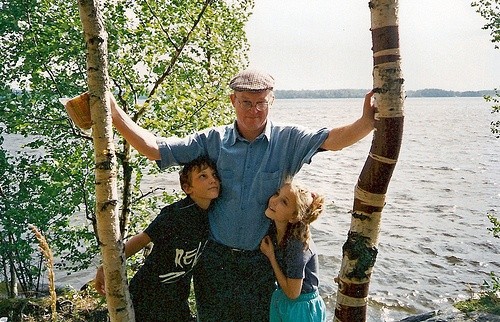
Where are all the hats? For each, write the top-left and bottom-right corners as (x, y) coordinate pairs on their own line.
(229, 70), (275, 92)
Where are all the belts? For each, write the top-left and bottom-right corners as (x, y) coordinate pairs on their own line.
(210, 241), (261, 258)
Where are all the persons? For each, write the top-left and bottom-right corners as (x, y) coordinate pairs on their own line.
(109, 70), (375, 322)
(260, 180), (326, 322)
(96, 155), (220, 322)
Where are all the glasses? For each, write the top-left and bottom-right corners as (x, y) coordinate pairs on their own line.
(236, 96), (271, 112)
